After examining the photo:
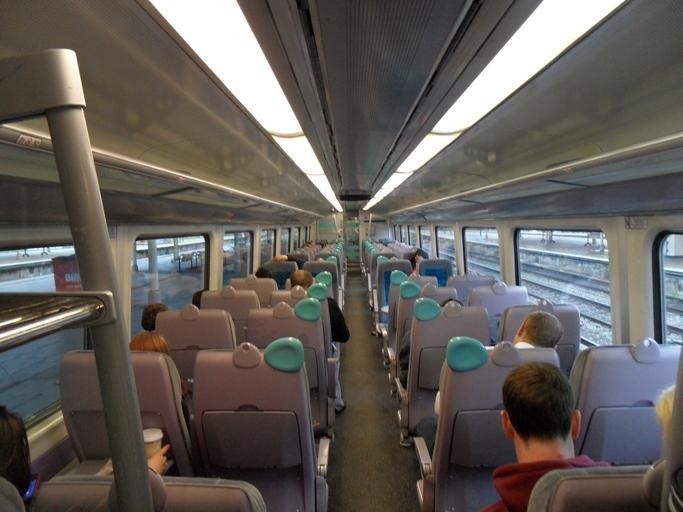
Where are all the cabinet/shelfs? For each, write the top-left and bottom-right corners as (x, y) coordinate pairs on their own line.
(0, 49), (153, 511)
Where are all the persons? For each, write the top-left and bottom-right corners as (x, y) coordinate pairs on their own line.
(141, 303), (171, 331)
(290, 270), (351, 343)
(410, 312), (562, 457)
(479, 363), (613, 508)
(127, 332), (189, 442)
(0, 406), (170, 505)
(254, 267), (273, 279)
(397, 298), (496, 390)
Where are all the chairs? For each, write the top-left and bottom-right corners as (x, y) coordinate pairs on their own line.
(59, 352), (194, 475)
(569, 338), (682, 464)
(200, 286), (260, 346)
(271, 283), (340, 359)
(1, 478), (25, 511)
(303, 257), (344, 305)
(414, 337), (559, 512)
(368, 253), (399, 307)
(155, 305), (235, 395)
(394, 298), (489, 436)
(387, 282), (457, 384)
(32, 477), (265, 512)
(373, 256), (412, 324)
(285, 271), (343, 312)
(194, 336), (330, 512)
(263, 258), (298, 290)
(231, 275), (278, 308)
(469, 282), (527, 341)
(419, 259), (452, 287)
(288, 240), (347, 273)
(361, 240), (418, 266)
(380, 270), (437, 357)
(248, 298), (336, 438)
(499, 300), (580, 374)
(527, 466), (659, 511)
(448, 272), (495, 306)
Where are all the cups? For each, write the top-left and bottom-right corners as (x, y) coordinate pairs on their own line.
(143, 427), (163, 459)
